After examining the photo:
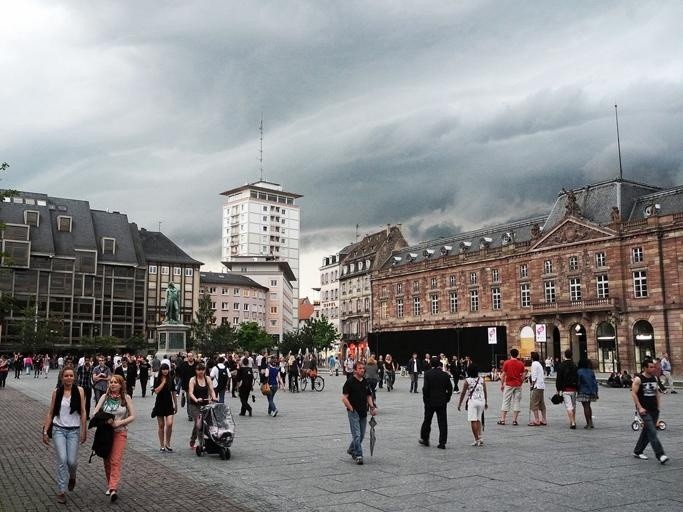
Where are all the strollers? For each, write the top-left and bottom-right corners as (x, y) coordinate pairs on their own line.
(196, 397), (234, 460)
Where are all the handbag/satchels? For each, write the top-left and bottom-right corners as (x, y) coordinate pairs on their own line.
(261, 383), (270, 394)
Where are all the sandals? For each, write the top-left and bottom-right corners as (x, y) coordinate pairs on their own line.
(497, 420), (505, 425)
(513, 421), (517, 425)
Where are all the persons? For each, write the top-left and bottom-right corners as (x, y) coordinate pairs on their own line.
(608, 352), (678, 465)
(328, 351), (488, 465)
(165, 281), (180, 322)
(91, 375), (136, 502)
(42, 366), (86, 499)
(497, 348), (599, 430)
(1, 347), (318, 452)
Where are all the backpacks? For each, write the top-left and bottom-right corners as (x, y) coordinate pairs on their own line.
(216, 365), (227, 387)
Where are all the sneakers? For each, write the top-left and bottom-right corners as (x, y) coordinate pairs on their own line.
(632, 452), (648, 460)
(268, 409), (278, 416)
(105, 489), (117, 502)
(239, 407), (252, 417)
(159, 446), (172, 452)
(660, 454), (669, 465)
(570, 421), (594, 429)
(347, 449), (363, 464)
(190, 435), (195, 446)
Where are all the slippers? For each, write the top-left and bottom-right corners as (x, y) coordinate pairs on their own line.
(528, 420), (546, 426)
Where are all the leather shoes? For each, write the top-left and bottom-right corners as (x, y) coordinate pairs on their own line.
(437, 444), (446, 449)
(419, 439), (429, 445)
(68, 478), (75, 490)
(57, 494), (67, 504)
(472, 437), (483, 446)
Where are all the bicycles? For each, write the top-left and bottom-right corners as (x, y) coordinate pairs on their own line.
(299, 369), (324, 392)
